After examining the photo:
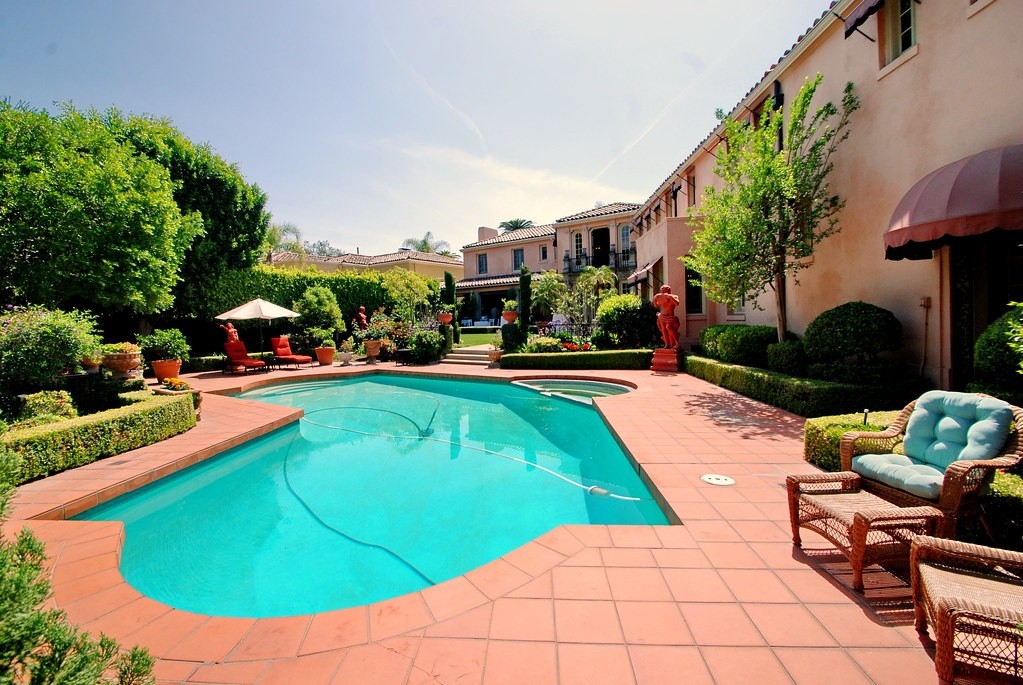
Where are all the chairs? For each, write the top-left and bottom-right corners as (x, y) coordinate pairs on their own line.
(840, 389), (1017, 539)
(271, 337), (314, 369)
(225, 342), (267, 376)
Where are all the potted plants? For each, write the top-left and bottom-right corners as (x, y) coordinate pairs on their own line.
(355, 320), (387, 365)
(289, 287), (348, 365)
(127, 355), (143, 380)
(501, 298), (518, 323)
(80, 346), (102, 371)
(135, 328), (192, 385)
(438, 303), (455, 326)
(104, 342), (141, 371)
(339, 336), (356, 366)
(529, 269), (568, 333)
(395, 336), (412, 366)
(489, 338), (504, 363)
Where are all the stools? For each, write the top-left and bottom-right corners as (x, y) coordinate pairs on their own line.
(786, 471), (948, 592)
(910, 535), (1023, 685)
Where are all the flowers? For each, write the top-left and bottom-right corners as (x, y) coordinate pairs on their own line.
(162, 377), (190, 390)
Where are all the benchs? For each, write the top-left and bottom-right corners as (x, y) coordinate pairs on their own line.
(474, 321), (489, 326)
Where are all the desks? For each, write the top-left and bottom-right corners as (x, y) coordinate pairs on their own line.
(461, 319), (472, 326)
(489, 319), (494, 326)
(480, 318), (488, 321)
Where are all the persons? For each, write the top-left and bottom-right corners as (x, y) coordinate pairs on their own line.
(653, 286), (680, 350)
(219, 323), (239, 342)
(357, 307), (370, 329)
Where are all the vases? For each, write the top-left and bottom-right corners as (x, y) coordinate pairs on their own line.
(152, 387), (202, 421)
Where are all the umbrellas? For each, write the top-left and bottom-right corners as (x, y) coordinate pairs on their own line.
(215, 298), (300, 358)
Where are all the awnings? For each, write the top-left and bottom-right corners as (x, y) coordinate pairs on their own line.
(881, 143), (1023, 262)
